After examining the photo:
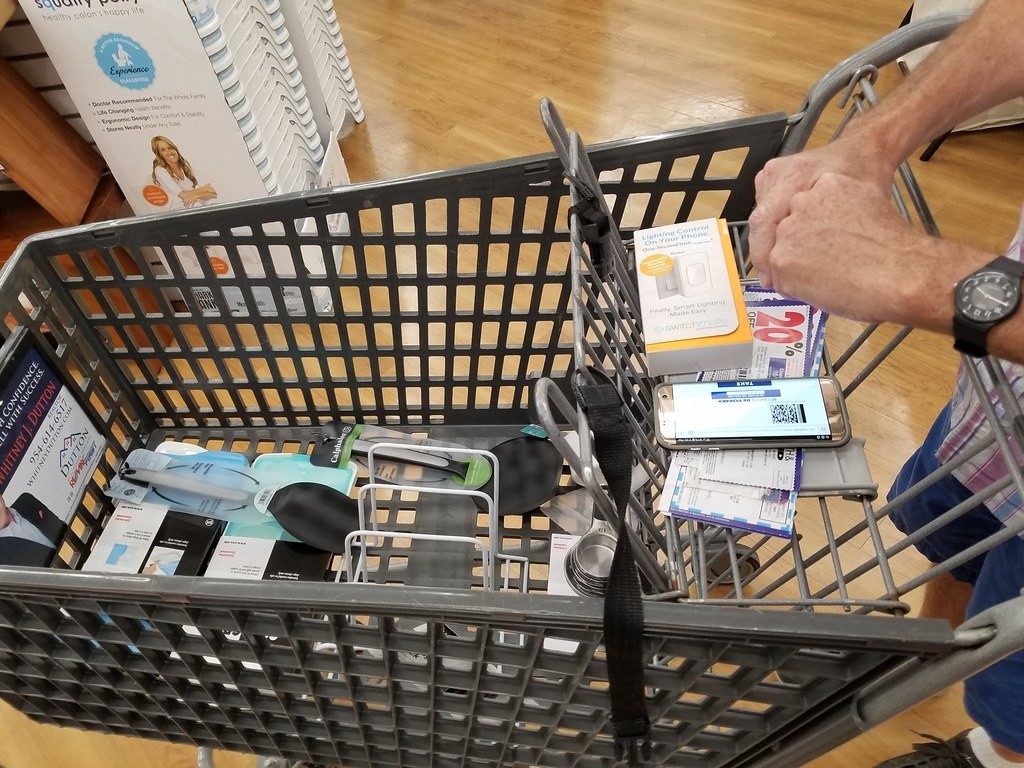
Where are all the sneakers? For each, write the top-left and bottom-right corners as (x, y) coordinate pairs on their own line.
(872, 728), (986, 768)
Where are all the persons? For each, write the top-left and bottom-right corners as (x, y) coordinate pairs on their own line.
(747, 0), (1024, 768)
(0, 491), (67, 567)
(150, 136), (220, 212)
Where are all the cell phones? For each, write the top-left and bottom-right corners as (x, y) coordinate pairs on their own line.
(653, 377), (851, 451)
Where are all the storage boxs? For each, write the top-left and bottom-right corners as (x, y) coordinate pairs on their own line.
(632, 215), (754, 378)
(19, 0), (351, 313)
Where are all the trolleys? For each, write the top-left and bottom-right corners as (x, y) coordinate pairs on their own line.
(0, 8), (1024, 768)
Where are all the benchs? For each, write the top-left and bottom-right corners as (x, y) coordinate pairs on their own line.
(0, 54), (107, 227)
(0, 174), (174, 373)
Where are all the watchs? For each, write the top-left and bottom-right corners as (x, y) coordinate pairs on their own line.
(950, 255), (1024, 358)
